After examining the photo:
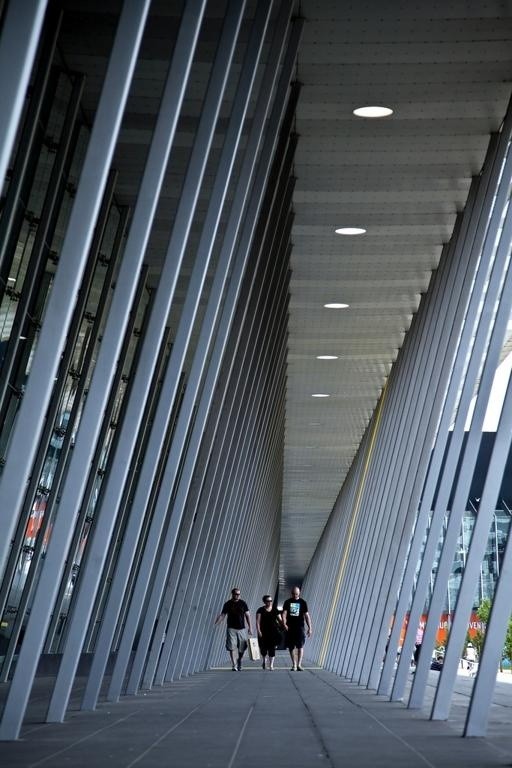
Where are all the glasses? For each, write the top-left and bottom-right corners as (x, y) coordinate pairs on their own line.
(265, 600), (272, 602)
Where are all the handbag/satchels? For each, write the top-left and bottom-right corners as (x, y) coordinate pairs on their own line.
(247, 637), (260, 661)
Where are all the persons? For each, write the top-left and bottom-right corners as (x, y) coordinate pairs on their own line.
(256, 595), (283, 671)
(215, 588), (252, 672)
(282, 587), (313, 672)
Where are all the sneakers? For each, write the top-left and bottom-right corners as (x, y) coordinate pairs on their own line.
(232, 659), (242, 671)
(291, 665), (304, 671)
